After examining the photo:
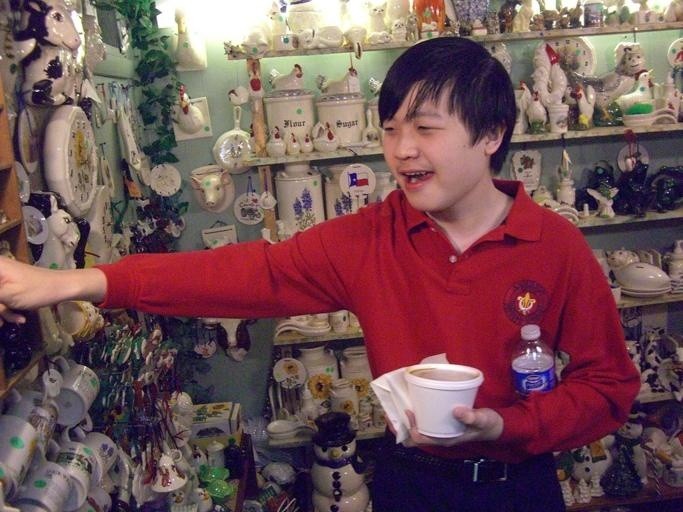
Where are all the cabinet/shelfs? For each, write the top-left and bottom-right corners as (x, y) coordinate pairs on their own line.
(227, 20), (683, 511)
(0, 79), (48, 396)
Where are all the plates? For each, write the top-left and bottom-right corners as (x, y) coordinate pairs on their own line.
(273, 358), (306, 390)
(617, 285), (673, 297)
(149, 164), (181, 198)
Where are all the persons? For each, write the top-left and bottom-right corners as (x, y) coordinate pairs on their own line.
(1, 35), (642, 511)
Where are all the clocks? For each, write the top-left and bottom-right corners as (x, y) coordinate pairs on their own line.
(43, 106), (98, 219)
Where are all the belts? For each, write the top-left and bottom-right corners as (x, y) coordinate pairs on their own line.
(389, 448), (556, 483)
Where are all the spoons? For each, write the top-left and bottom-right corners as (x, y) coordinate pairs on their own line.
(622, 115), (678, 127)
(623, 107), (676, 120)
(274, 326), (331, 338)
(274, 319), (330, 330)
(266, 420), (319, 434)
(267, 428), (313, 440)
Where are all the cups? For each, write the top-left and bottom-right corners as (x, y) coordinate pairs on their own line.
(60, 425), (119, 478)
(48, 355), (101, 427)
(548, 103), (570, 133)
(49, 437), (95, 504)
(403, 364), (484, 440)
(5, 388), (60, 457)
(17, 443), (72, 512)
(0, 414), (38, 502)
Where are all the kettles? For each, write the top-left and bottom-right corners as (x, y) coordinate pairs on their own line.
(207, 440), (225, 468)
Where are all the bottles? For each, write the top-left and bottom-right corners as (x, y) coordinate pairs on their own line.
(511, 324), (558, 400)
(299, 345), (375, 430)
